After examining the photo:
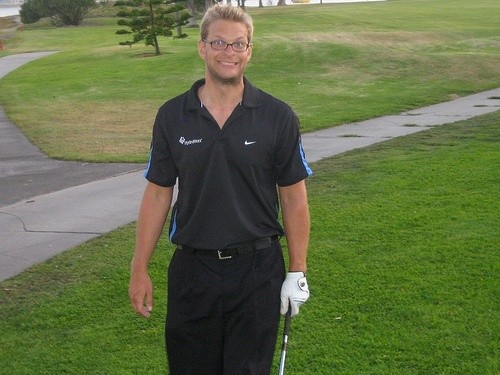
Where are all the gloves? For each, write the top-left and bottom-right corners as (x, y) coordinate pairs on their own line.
(280, 271), (310, 318)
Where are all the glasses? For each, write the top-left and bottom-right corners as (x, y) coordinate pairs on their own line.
(202, 39), (252, 52)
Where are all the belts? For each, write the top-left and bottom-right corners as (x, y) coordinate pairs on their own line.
(177, 235), (279, 259)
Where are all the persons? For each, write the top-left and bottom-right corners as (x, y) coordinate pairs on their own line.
(130, 3), (312, 375)
